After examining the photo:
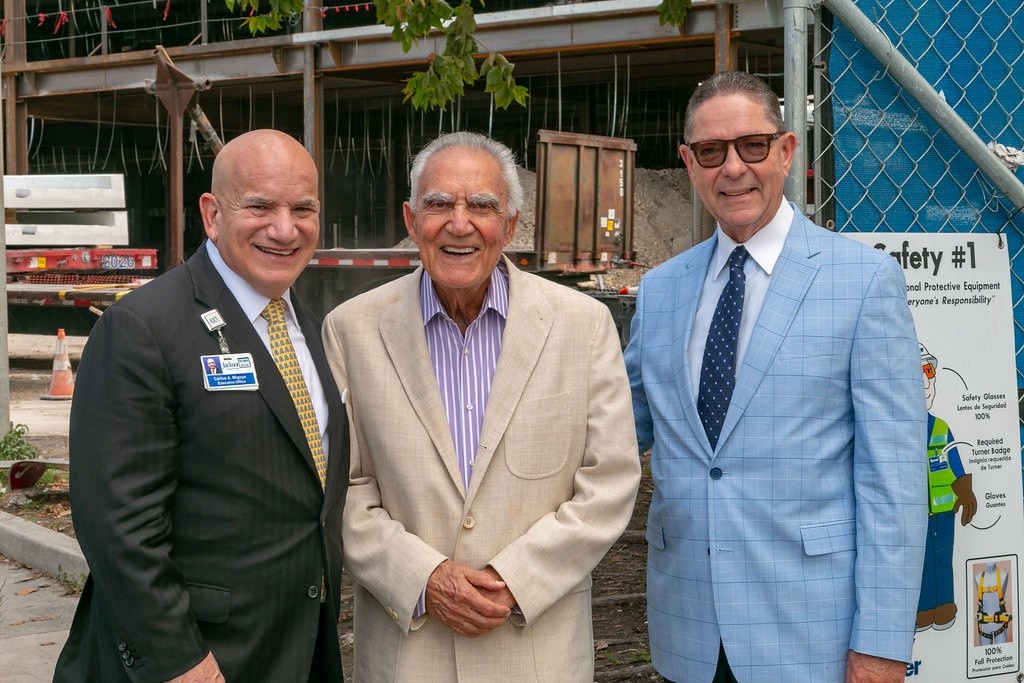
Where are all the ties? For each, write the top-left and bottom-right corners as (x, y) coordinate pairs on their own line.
(260, 297), (327, 604)
(696, 245), (750, 452)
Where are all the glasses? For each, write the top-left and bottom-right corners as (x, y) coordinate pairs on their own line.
(683, 133), (779, 168)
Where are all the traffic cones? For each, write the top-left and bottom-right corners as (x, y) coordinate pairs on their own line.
(39, 327), (76, 401)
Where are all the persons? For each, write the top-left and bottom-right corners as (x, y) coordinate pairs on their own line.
(51, 128), (350, 683)
(623, 70), (930, 683)
(206, 357), (223, 374)
(321, 130), (642, 683)
